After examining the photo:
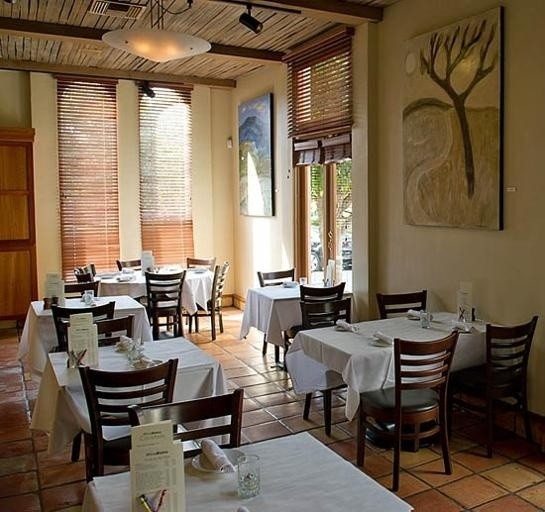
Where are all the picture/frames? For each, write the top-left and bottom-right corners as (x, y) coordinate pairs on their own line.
(239, 92), (274, 216)
(401, 6), (502, 229)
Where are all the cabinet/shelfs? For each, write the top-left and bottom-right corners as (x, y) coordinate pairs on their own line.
(1, 127), (38, 320)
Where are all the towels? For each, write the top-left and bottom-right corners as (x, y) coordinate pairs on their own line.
(335, 318), (359, 334)
(407, 308), (433, 321)
(133, 352), (162, 368)
(373, 330), (394, 345)
(200, 437), (235, 474)
(283, 280), (298, 287)
(450, 321), (475, 332)
(84, 292), (96, 306)
(113, 334), (143, 353)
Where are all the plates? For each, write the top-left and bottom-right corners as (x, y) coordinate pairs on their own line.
(368, 338), (393, 348)
(191, 448), (247, 474)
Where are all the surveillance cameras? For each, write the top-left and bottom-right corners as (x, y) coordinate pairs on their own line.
(238, 12), (263, 34)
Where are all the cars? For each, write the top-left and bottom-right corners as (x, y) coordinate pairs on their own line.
(311, 235), (351, 272)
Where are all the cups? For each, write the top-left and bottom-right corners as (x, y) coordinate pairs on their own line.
(128, 338), (141, 362)
(299, 277), (308, 285)
(84, 289), (94, 305)
(236, 453), (262, 499)
(419, 310), (431, 329)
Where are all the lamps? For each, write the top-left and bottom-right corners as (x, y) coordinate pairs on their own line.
(102, 0), (212, 63)
(239, 4), (263, 33)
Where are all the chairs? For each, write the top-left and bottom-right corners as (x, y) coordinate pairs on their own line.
(187, 258), (216, 272)
(283, 283), (346, 339)
(51, 301), (116, 352)
(77, 358), (179, 482)
(116, 260), (142, 271)
(257, 269), (294, 361)
(75, 264), (96, 296)
(128, 388), (243, 458)
(240, 283), (360, 361)
(301, 298), (352, 435)
(357, 330), (459, 491)
(377, 290), (427, 319)
(449, 316), (538, 457)
(143, 271), (186, 341)
(65, 281), (100, 298)
(62, 315), (137, 460)
(182, 261), (229, 341)
